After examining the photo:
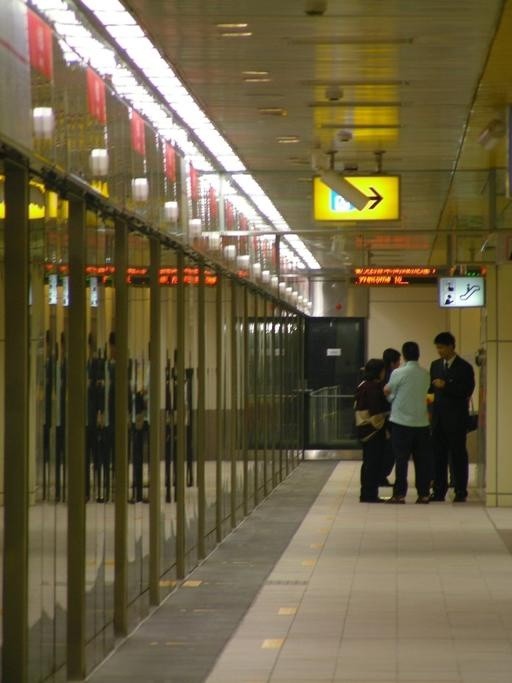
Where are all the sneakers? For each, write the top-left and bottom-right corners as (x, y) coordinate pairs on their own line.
(384, 496), (405, 503)
(416, 496), (429, 503)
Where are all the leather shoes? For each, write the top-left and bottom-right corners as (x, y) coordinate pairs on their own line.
(429, 493), (444, 501)
(454, 490), (467, 500)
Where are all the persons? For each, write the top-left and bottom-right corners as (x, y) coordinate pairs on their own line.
(425, 332), (475, 503)
(355, 358), (390, 502)
(384, 341), (434, 505)
(377, 348), (400, 486)
